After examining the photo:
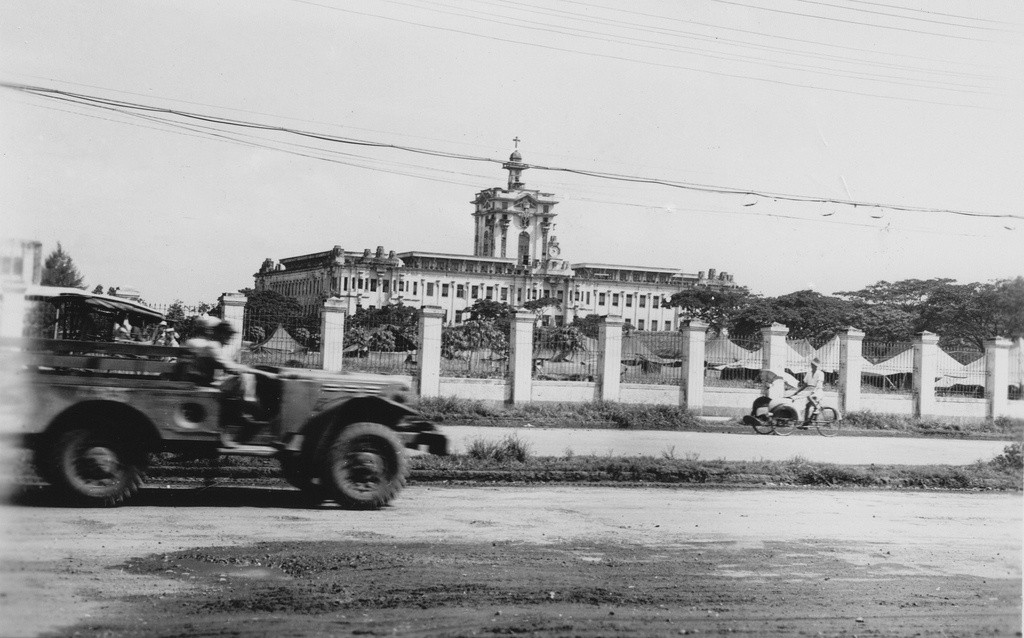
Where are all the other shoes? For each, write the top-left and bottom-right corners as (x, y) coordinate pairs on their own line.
(797, 425), (809, 431)
(812, 407), (822, 414)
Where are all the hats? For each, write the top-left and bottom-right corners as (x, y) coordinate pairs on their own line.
(165, 328), (174, 332)
(764, 367), (784, 379)
(809, 358), (821, 366)
(213, 322), (237, 335)
(193, 316), (223, 329)
(159, 321), (168, 326)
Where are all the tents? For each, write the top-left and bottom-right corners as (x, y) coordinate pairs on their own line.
(556, 327), (1024, 394)
(250, 324), (305, 355)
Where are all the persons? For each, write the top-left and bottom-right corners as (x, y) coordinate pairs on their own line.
(110, 314), (278, 422)
(796, 358), (824, 430)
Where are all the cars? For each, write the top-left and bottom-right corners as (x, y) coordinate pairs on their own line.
(0, 287), (449, 509)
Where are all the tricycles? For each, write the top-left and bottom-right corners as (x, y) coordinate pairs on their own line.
(742, 383), (842, 438)
